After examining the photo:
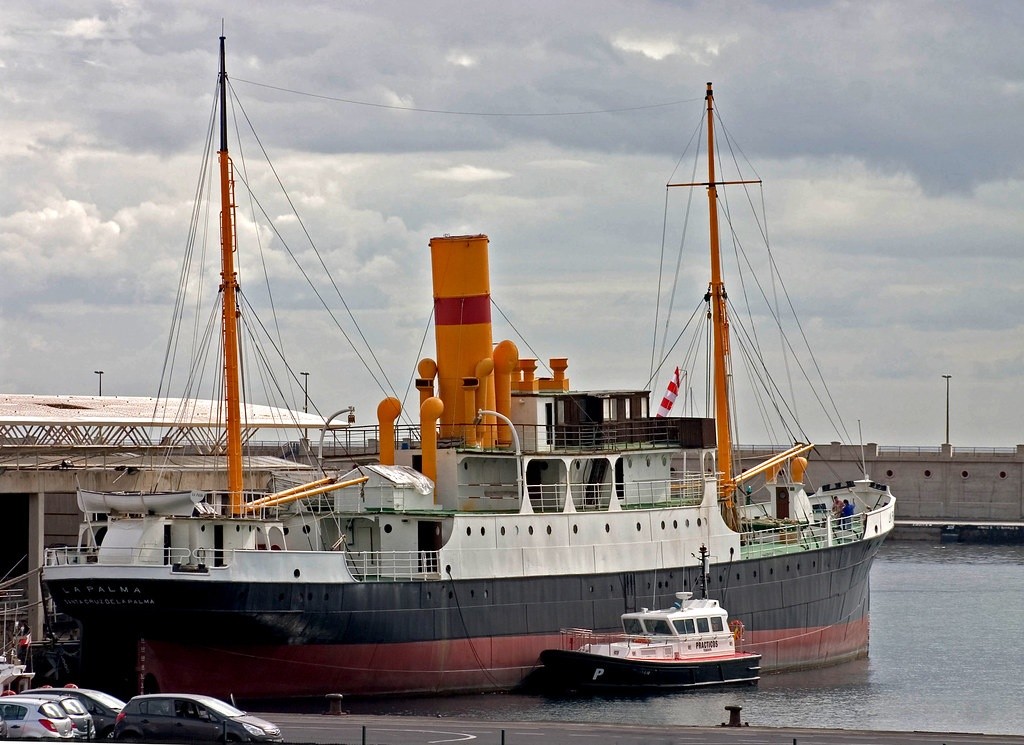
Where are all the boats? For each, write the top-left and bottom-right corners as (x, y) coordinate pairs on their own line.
(34, 20), (902, 710)
(538, 544), (764, 688)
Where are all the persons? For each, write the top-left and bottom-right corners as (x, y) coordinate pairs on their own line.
(832, 496), (856, 530)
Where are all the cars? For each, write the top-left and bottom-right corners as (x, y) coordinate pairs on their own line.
(1, 694), (97, 742)
(115, 693), (284, 745)
(20, 687), (127, 742)
(0, 698), (77, 741)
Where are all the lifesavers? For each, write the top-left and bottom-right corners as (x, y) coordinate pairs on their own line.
(728, 620), (743, 639)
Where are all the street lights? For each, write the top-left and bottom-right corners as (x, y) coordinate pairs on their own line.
(93, 371), (104, 397)
(940, 373), (953, 444)
(299, 371), (310, 438)
